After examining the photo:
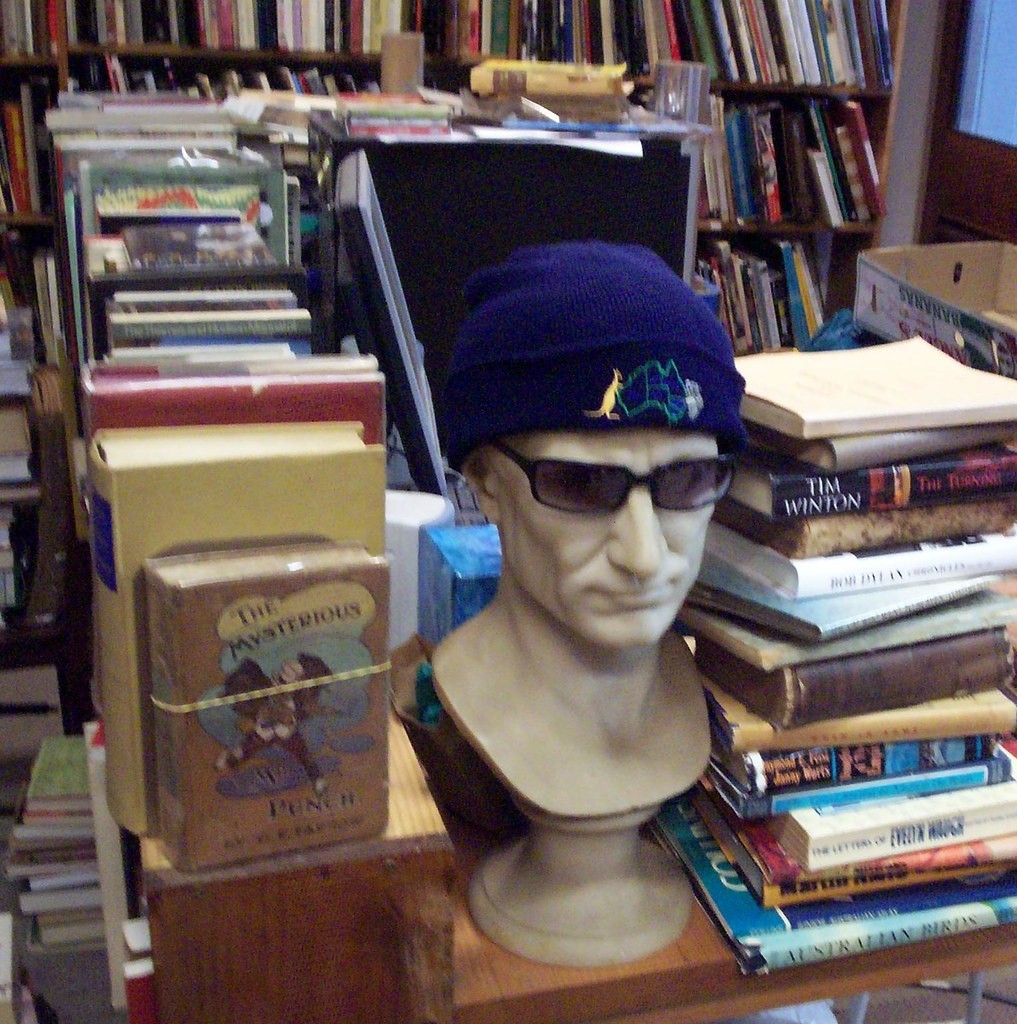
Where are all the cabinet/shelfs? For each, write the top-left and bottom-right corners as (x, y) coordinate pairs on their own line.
(0, 0), (1017, 1024)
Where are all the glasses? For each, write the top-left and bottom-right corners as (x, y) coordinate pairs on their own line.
(489, 439), (735, 514)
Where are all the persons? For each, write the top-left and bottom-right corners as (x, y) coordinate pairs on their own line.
(430, 241), (752, 967)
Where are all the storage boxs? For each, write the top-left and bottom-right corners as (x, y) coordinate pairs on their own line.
(310, 107), (713, 490)
(855, 241), (1017, 382)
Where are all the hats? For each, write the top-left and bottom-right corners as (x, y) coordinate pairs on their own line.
(442, 237), (746, 475)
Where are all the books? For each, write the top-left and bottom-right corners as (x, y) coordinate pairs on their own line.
(0, 0), (1017, 1021)
